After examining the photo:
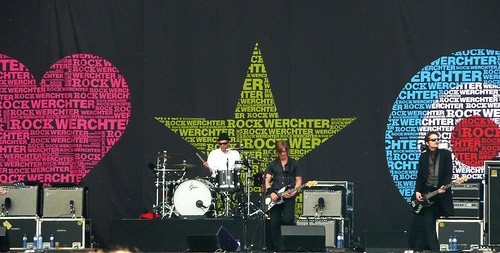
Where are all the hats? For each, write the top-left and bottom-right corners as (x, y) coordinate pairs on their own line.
(217, 133), (230, 141)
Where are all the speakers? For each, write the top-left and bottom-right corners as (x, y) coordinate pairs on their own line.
(0, 226), (9, 252)
(186, 224), (239, 253)
(280, 225), (325, 252)
(40, 185), (88, 218)
(488, 166), (500, 247)
(302, 186), (346, 217)
(0, 184), (41, 216)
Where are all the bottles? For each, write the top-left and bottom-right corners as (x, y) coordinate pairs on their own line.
(449, 236), (453, 251)
(452, 236), (457, 250)
(337, 233), (342, 248)
(23, 234), (27, 248)
(50, 234), (55, 249)
(38, 234), (42, 248)
(33, 237), (37, 248)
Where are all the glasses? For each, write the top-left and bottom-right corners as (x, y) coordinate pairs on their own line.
(428, 137), (440, 142)
(219, 142), (227, 145)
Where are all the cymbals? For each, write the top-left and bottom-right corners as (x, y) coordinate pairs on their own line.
(151, 151), (186, 158)
(229, 147), (260, 150)
(170, 163), (197, 169)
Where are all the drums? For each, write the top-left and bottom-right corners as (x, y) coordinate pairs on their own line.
(172, 178), (219, 215)
(215, 169), (241, 192)
(153, 168), (182, 186)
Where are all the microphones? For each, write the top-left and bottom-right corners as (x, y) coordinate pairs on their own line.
(70, 200), (74, 213)
(253, 159), (264, 163)
(319, 198), (324, 213)
(5, 198), (11, 214)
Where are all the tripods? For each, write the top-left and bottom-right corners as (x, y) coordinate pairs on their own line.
(154, 151), (270, 220)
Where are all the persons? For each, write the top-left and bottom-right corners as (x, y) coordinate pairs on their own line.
(265, 140), (302, 253)
(203, 132), (242, 181)
(410, 132), (454, 253)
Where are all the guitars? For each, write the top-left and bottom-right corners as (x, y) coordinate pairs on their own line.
(261, 180), (319, 213)
(410, 175), (469, 215)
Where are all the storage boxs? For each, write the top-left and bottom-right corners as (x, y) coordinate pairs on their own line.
(436, 219), (484, 252)
(0, 217), (92, 250)
